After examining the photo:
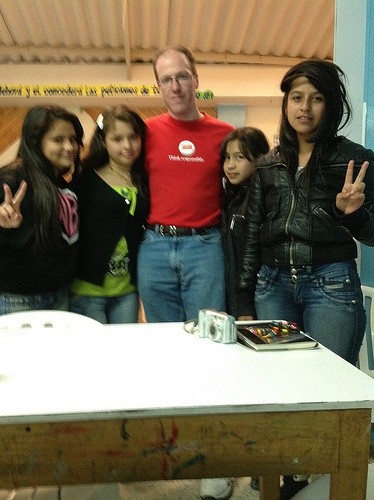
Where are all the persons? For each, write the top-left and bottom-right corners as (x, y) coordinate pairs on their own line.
(237, 58), (374, 500)
(68, 103), (150, 322)
(0, 102), (84, 314)
(133, 43), (240, 500)
(220, 127), (270, 319)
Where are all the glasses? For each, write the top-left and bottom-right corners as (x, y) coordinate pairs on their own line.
(159, 72), (195, 90)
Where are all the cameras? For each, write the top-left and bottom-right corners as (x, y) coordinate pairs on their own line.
(198, 309), (237, 344)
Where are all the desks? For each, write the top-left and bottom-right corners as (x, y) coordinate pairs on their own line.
(1, 319), (374, 500)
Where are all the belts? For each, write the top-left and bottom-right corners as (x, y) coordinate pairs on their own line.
(143, 222), (221, 239)
